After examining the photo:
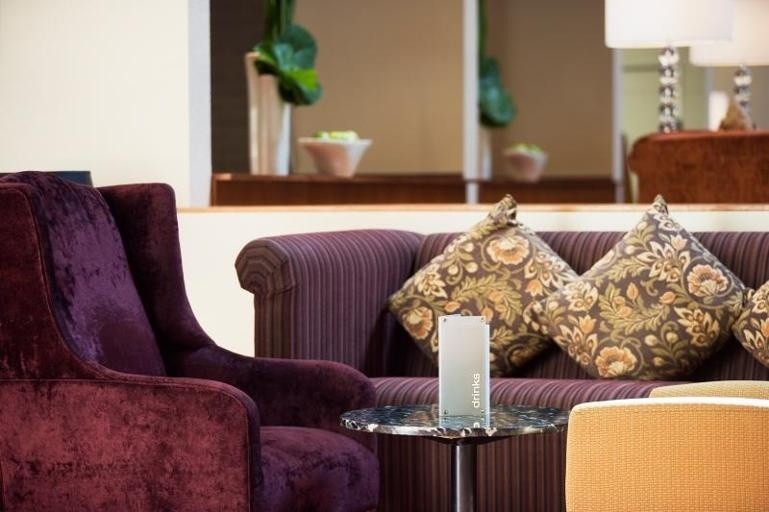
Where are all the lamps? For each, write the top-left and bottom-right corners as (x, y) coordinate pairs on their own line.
(601, 0), (714, 138)
(690, 3), (768, 136)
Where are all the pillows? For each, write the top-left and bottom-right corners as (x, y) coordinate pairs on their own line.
(523, 193), (754, 379)
(385, 192), (588, 377)
(728, 278), (769, 374)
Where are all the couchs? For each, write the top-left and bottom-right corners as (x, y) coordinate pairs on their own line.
(225, 222), (768, 417)
(559, 378), (769, 511)
(0, 167), (381, 509)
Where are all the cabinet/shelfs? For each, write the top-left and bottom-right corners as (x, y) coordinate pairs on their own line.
(627, 128), (768, 207)
(208, 167), (622, 210)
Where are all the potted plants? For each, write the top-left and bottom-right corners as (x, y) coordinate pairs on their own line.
(294, 126), (375, 181)
(239, 1), (323, 178)
(501, 142), (551, 181)
